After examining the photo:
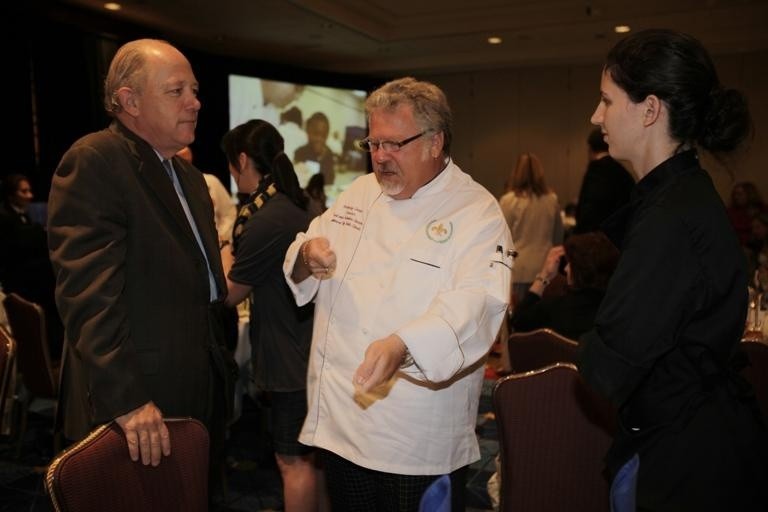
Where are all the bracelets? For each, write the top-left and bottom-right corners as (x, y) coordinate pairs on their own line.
(535, 273), (550, 286)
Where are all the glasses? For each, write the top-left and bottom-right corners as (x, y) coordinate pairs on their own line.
(359, 128), (434, 150)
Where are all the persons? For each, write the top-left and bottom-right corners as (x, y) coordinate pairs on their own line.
(277, 106), (308, 161)
(47, 38), (238, 512)
(726, 181), (768, 248)
(225, 120), (329, 511)
(499, 153), (562, 304)
(575, 130), (636, 235)
(229, 75), (363, 204)
(176, 147), (236, 276)
(1, 174), (64, 363)
(574, 29), (768, 512)
(509, 231), (620, 342)
(303, 175), (329, 221)
(294, 113), (335, 185)
(282, 76), (518, 511)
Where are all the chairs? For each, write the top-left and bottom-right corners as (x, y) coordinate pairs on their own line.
(44, 413), (210, 512)
(0, 323), (16, 418)
(507, 327), (579, 370)
(492, 360), (614, 511)
(1, 292), (62, 457)
